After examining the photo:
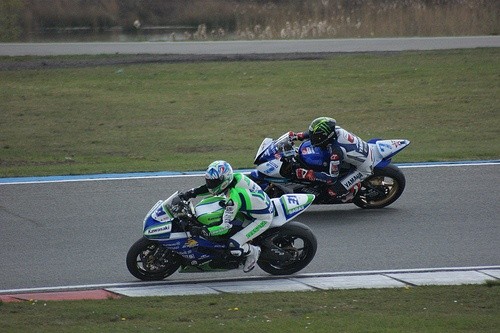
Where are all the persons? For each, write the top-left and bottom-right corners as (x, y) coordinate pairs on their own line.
(171, 160), (276, 273)
(286, 117), (375, 202)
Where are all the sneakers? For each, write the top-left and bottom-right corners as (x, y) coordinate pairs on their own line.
(243, 244), (261, 272)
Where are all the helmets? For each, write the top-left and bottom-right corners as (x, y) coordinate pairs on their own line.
(309, 117), (336, 147)
(204, 159), (233, 195)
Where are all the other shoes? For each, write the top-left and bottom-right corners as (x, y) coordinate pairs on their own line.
(341, 183), (361, 203)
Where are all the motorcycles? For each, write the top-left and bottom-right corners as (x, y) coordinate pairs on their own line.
(126, 191), (318, 281)
(242, 133), (410, 211)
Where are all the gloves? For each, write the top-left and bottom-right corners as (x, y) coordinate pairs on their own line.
(289, 131), (304, 141)
(190, 226), (211, 238)
(172, 192), (189, 206)
(296, 168), (314, 182)
